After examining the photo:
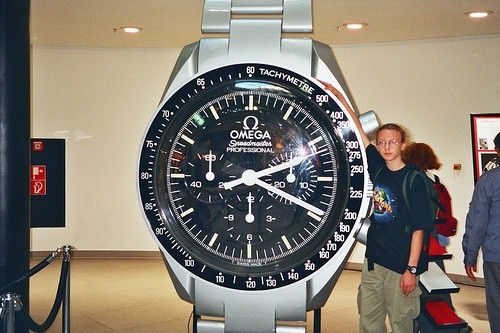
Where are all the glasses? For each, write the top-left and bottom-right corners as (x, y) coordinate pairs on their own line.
(376, 140), (401, 147)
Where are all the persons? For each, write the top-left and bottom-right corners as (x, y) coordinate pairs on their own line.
(461, 131), (500, 333)
(402, 140), (446, 274)
(316, 78), (431, 333)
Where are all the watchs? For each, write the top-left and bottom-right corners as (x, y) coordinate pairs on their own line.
(404, 265), (417, 275)
(135, 1), (383, 333)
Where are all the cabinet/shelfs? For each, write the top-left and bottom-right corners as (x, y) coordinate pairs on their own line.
(414, 219), (468, 333)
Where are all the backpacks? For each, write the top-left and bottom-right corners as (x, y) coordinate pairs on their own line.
(427, 174), (458, 237)
